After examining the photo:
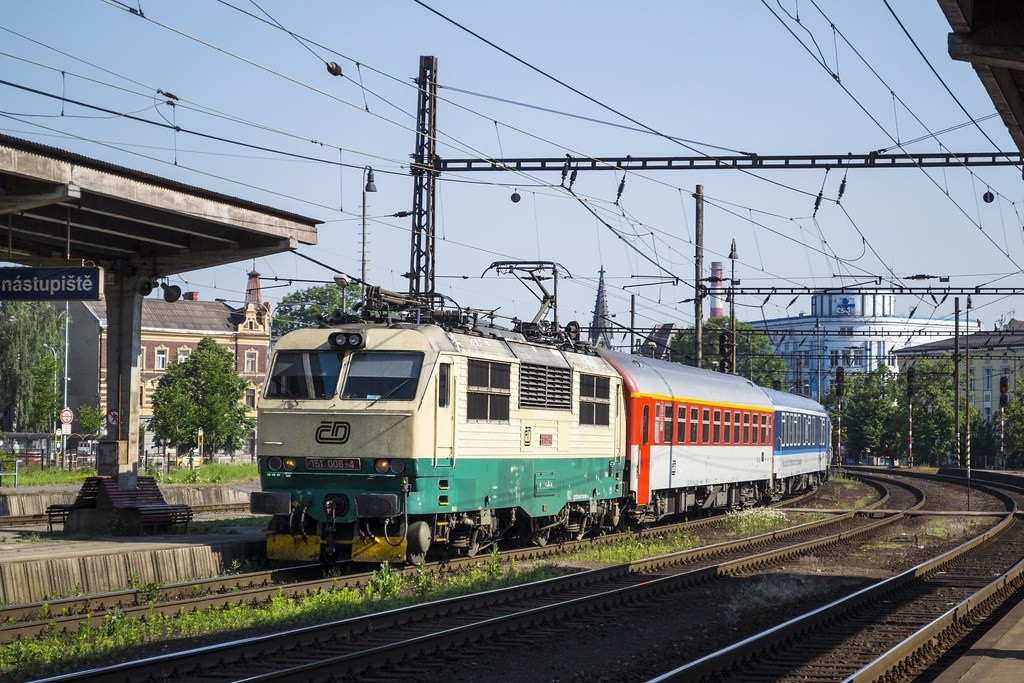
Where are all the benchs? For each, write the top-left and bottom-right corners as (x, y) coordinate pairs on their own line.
(45, 476), (98, 533)
(97, 476), (194, 537)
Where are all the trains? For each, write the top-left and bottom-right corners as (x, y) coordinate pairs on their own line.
(246, 260), (834, 559)
